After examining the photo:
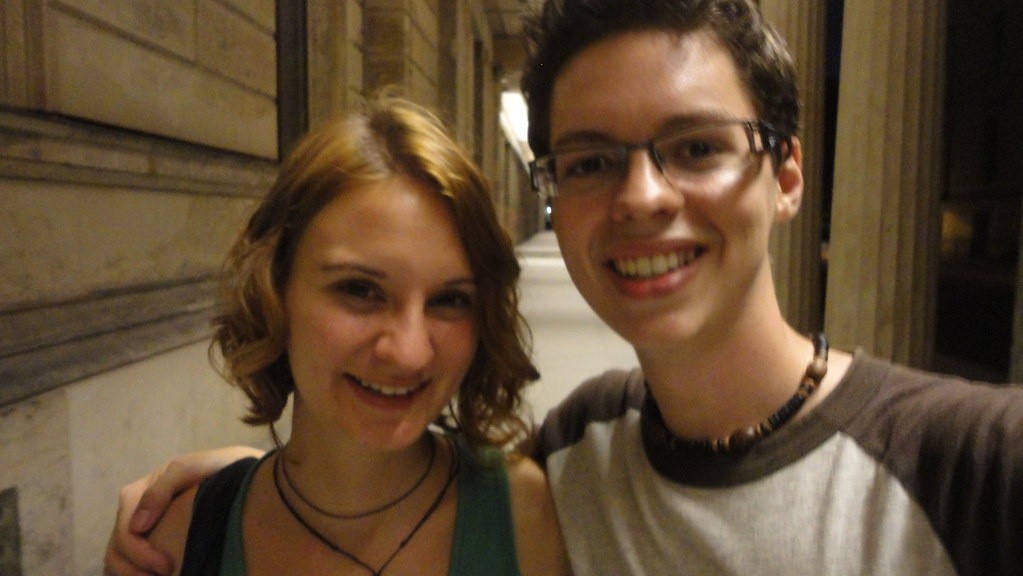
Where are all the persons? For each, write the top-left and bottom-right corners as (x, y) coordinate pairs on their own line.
(105, 0), (1022, 576)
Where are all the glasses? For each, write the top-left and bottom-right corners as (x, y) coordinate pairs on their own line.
(529, 120), (791, 198)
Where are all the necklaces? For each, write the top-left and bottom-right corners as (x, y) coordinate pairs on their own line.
(644, 331), (830, 455)
(282, 430), (435, 520)
(274, 434), (460, 576)
